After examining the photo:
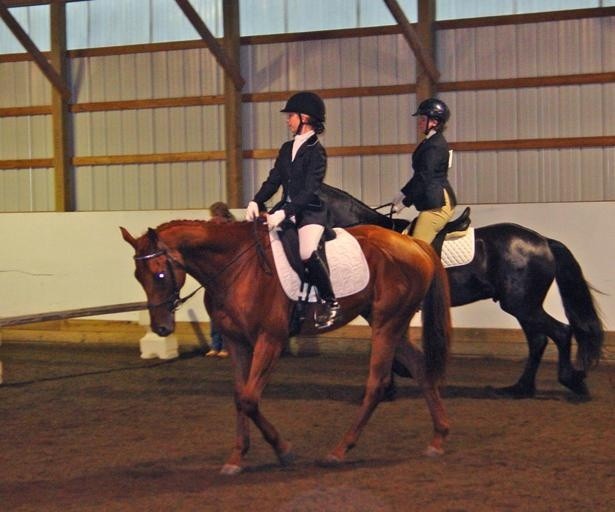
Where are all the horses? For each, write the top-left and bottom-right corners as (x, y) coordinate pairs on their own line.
(319, 183), (609, 402)
(118, 220), (452, 477)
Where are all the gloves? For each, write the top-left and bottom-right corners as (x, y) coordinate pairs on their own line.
(392, 191), (406, 213)
(267, 209), (286, 231)
(246, 201), (260, 223)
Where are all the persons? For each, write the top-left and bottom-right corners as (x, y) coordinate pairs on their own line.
(245, 90), (344, 331)
(392, 98), (457, 245)
(205, 202), (236, 359)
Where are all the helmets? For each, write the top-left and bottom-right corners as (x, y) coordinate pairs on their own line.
(412, 98), (450, 122)
(280, 92), (326, 121)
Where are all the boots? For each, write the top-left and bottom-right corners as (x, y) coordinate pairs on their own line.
(304, 250), (344, 331)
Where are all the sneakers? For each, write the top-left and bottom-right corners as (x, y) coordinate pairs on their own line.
(218, 352), (229, 358)
(205, 350), (217, 356)
